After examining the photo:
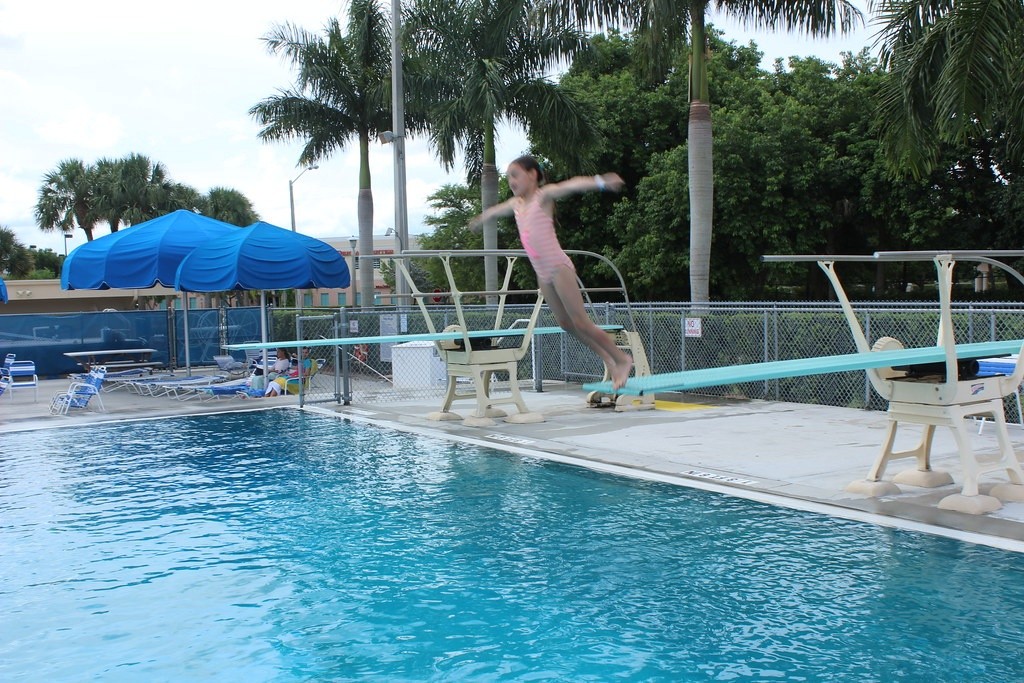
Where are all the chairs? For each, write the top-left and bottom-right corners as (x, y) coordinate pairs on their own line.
(436, 372), (499, 386)
(0, 354), (39, 404)
(47, 340), (326, 417)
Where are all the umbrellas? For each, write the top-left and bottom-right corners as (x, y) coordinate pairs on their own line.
(60, 209), (246, 379)
(174, 221), (351, 391)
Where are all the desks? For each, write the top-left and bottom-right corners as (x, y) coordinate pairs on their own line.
(64, 348), (161, 377)
(392, 341), (442, 388)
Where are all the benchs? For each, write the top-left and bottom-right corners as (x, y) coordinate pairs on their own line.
(77, 359), (148, 366)
(90, 362), (163, 368)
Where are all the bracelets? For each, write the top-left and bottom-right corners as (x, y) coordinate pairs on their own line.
(592, 173), (606, 190)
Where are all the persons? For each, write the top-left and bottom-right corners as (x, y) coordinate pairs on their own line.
(468, 155), (632, 391)
(248, 346), (312, 397)
